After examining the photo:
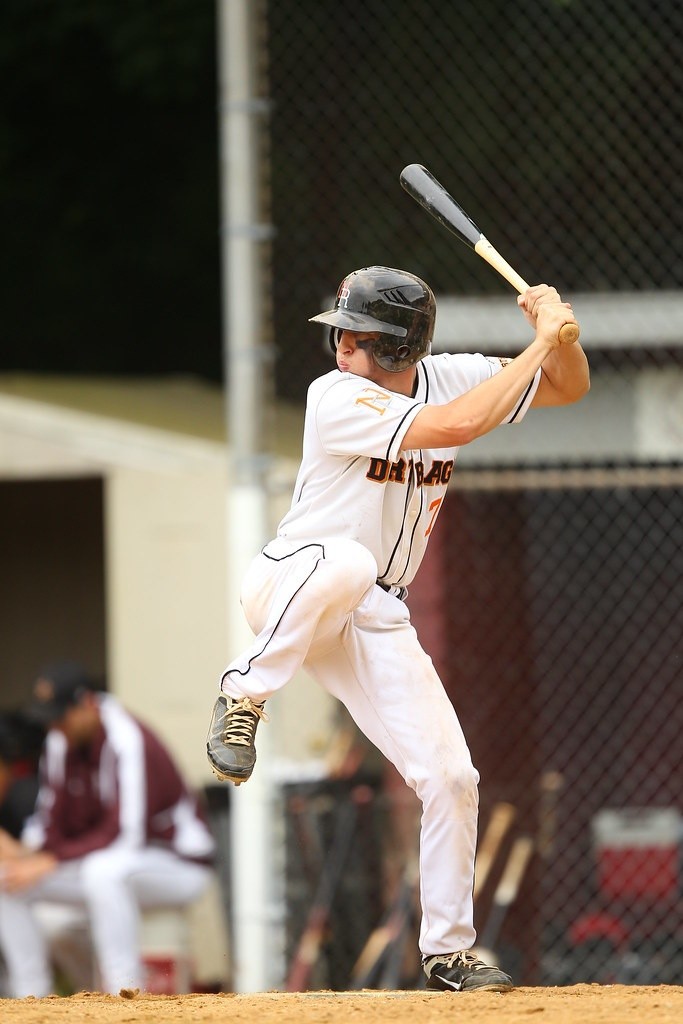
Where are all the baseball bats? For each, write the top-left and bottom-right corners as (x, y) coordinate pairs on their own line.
(398, 166), (580, 344)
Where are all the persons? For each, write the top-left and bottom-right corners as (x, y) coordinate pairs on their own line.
(1, 658), (218, 997)
(205, 266), (591, 990)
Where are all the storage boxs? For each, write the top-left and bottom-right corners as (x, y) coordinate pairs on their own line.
(591, 808), (682, 911)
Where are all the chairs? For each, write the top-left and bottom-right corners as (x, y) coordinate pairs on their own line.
(85, 785), (234, 994)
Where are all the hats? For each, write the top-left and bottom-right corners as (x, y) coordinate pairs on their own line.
(22, 671), (84, 719)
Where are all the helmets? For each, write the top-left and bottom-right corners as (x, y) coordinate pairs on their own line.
(306, 265), (436, 373)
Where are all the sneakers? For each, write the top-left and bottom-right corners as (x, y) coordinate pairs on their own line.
(420, 949), (515, 992)
(204, 693), (269, 788)
